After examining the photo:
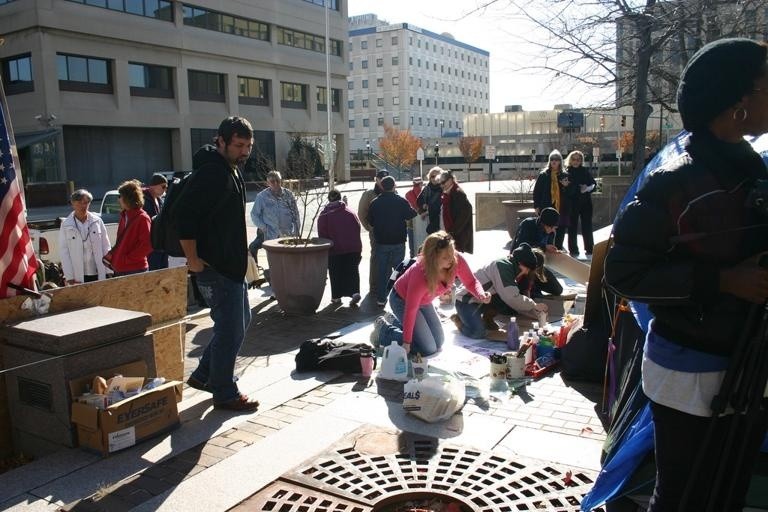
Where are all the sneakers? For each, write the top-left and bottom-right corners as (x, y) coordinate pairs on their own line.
(487, 329), (508, 342)
(383, 312), (395, 324)
(349, 295), (360, 305)
(450, 314), (463, 330)
(369, 316), (386, 349)
(331, 298), (340, 303)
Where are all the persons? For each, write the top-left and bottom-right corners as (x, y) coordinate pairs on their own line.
(510, 207), (563, 298)
(370, 229), (492, 356)
(58, 189), (114, 285)
(405, 166), (474, 259)
(449, 242), (563, 340)
(563, 150), (597, 260)
(102, 179), (153, 276)
(359, 170), (417, 309)
(604, 37), (768, 512)
(533, 149), (574, 253)
(173, 115), (260, 411)
(142, 174), (168, 271)
(318, 189), (362, 304)
(644, 145), (654, 165)
(248, 170), (300, 262)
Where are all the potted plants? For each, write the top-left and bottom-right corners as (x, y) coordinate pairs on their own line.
(494, 143), (549, 240)
(243, 117), (348, 318)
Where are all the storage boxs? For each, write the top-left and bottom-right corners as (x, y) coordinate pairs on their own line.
(68, 359), (184, 459)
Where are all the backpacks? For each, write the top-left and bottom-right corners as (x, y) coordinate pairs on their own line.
(294, 334), (377, 373)
(150, 163), (234, 257)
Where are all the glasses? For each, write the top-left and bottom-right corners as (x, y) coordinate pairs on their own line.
(546, 224), (558, 229)
(572, 157), (582, 162)
(549, 157), (560, 161)
(437, 179), (447, 186)
(159, 184), (165, 189)
(436, 234), (451, 249)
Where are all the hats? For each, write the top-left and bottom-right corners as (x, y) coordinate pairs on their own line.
(676, 37), (762, 132)
(149, 173), (167, 186)
(539, 207), (560, 226)
(512, 242), (536, 270)
(376, 170), (388, 178)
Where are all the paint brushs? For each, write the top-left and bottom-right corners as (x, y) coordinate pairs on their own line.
(515, 339), (533, 358)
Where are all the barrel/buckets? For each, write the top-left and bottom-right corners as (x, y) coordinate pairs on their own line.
(381, 341), (408, 381)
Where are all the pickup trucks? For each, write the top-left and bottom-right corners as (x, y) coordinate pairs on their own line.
(26, 173), (171, 275)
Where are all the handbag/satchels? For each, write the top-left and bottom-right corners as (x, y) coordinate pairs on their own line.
(386, 256), (417, 292)
(102, 250), (113, 270)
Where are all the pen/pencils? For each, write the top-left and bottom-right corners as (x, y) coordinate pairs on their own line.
(489, 352), (506, 364)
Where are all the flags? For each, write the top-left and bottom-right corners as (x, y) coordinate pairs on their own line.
(1, 79), (40, 299)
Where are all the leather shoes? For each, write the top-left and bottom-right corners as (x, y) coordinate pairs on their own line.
(213, 395), (259, 412)
(186, 376), (239, 393)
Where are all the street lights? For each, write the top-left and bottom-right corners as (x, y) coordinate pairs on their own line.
(434, 141), (440, 167)
(366, 141), (370, 159)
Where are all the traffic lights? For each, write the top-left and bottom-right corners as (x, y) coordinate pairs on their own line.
(569, 112), (575, 128)
(622, 114), (627, 127)
